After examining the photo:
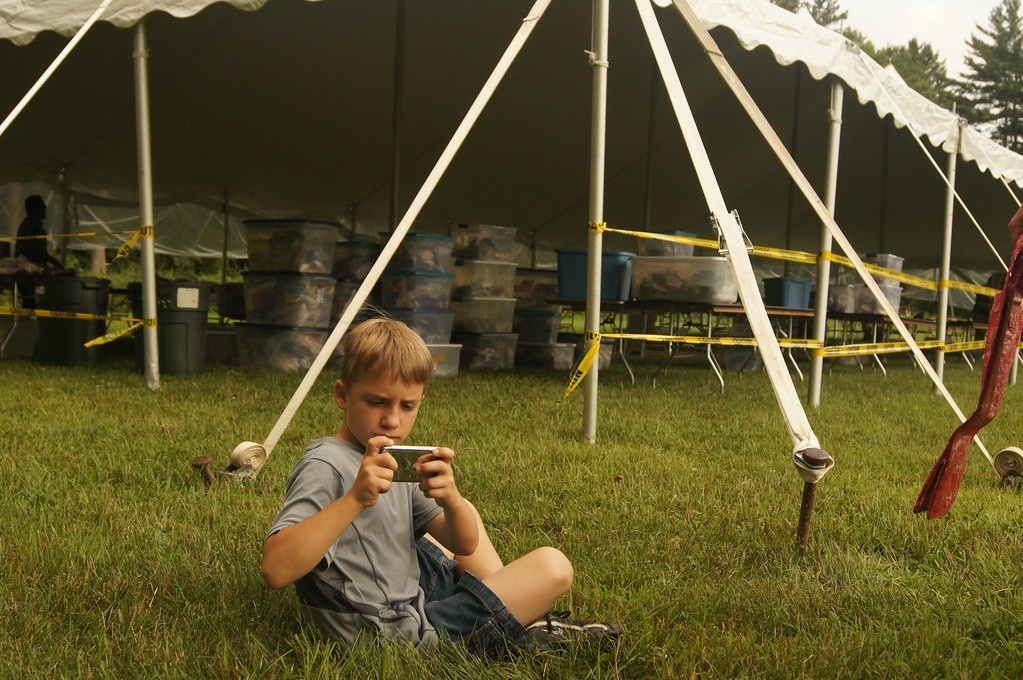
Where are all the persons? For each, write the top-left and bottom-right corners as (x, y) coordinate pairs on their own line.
(12, 193), (57, 316)
(261, 319), (614, 662)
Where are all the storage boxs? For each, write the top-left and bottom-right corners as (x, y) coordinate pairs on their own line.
(553, 248), (637, 302)
(762, 277), (812, 308)
(637, 230), (698, 257)
(206, 218), (614, 376)
(721, 346), (765, 373)
(809, 253), (905, 315)
(631, 256), (738, 304)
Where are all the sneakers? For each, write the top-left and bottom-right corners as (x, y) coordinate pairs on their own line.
(527, 611), (623, 651)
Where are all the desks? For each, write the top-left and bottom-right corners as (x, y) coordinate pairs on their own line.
(544, 298), (1023, 395)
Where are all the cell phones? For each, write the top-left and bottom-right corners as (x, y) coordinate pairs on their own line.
(381, 445), (439, 483)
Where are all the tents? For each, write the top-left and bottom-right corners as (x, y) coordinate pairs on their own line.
(0, 1), (1022, 445)
